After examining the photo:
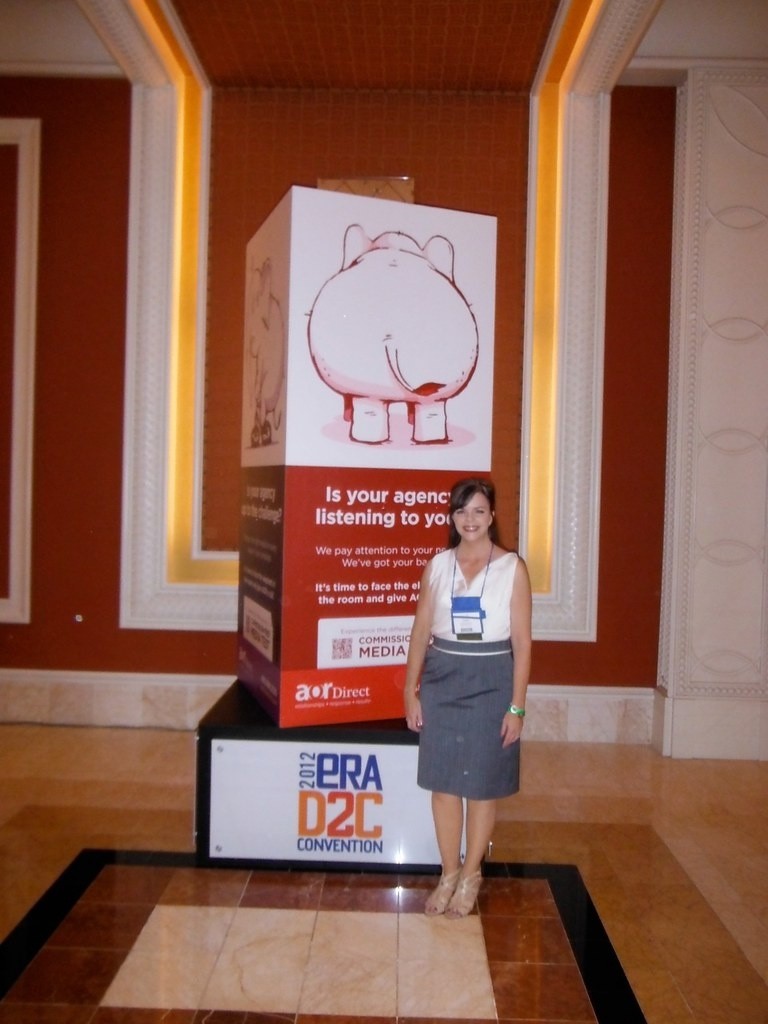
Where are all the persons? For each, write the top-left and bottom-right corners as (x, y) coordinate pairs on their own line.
(402, 478), (532, 920)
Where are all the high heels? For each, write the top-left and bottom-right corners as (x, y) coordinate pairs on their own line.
(424, 862), (463, 916)
(446, 866), (482, 919)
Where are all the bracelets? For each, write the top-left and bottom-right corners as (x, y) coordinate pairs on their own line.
(507, 705), (526, 718)
